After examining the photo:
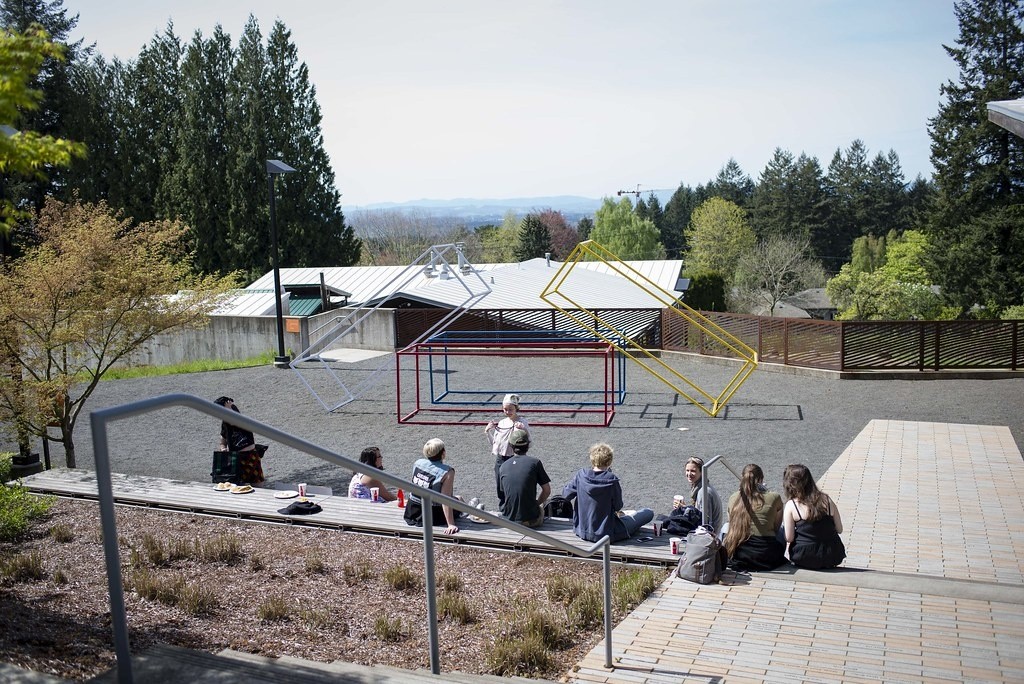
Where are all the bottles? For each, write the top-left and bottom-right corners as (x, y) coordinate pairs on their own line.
(397, 488), (404, 507)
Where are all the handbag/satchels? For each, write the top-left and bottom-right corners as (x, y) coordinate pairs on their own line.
(255, 443), (268, 458)
(212, 450), (241, 483)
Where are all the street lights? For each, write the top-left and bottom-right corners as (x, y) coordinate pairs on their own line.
(264, 158), (298, 370)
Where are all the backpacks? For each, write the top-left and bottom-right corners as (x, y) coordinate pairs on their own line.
(543, 496), (574, 519)
(673, 524), (728, 584)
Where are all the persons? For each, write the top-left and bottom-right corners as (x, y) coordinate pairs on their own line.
(499, 429), (551, 527)
(562, 444), (654, 543)
(783, 463), (847, 568)
(722, 464), (784, 571)
(404, 437), (485, 535)
(348, 447), (397, 503)
(213, 396), (266, 488)
(485, 394), (533, 506)
(656, 456), (722, 535)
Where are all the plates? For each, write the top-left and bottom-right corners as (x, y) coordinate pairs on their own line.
(466, 513), (498, 523)
(213, 484), (237, 490)
(229, 486), (254, 493)
(274, 490), (298, 498)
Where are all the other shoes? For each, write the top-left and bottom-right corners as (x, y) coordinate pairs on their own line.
(459, 497), (485, 518)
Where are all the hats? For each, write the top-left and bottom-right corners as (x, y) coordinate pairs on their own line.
(277, 501), (323, 515)
(503, 394), (521, 405)
(509, 430), (532, 446)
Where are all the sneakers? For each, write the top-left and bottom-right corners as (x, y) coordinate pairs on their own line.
(617, 509), (637, 518)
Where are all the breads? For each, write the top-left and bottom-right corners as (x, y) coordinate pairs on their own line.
(218, 481), (231, 488)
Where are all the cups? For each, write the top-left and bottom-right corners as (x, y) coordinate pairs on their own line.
(654, 521), (663, 537)
(669, 537), (681, 554)
(673, 495), (684, 509)
(370, 487), (379, 500)
(298, 482), (307, 496)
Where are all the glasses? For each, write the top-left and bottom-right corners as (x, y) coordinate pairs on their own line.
(376, 455), (381, 459)
